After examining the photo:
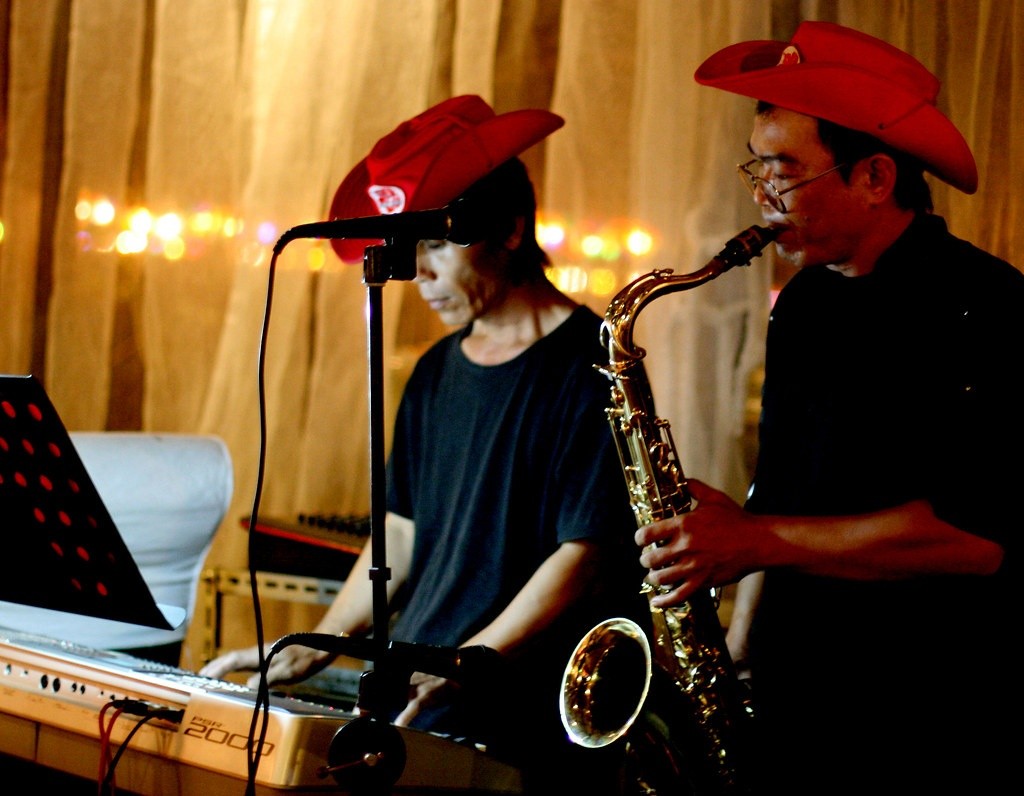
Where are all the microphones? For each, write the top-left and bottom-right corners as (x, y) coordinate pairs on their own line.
(273, 633), (507, 689)
(290, 195), (488, 247)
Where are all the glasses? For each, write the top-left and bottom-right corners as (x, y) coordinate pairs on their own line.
(733, 157), (862, 215)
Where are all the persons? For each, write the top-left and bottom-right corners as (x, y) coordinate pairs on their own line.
(201, 95), (656, 795)
(632, 15), (1023, 795)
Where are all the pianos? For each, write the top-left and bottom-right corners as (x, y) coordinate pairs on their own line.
(0, 626), (528, 796)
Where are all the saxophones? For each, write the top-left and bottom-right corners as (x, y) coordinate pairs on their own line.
(557, 217), (788, 795)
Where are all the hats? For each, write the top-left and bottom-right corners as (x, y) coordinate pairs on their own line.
(694, 21), (979, 196)
(329, 94), (565, 265)
